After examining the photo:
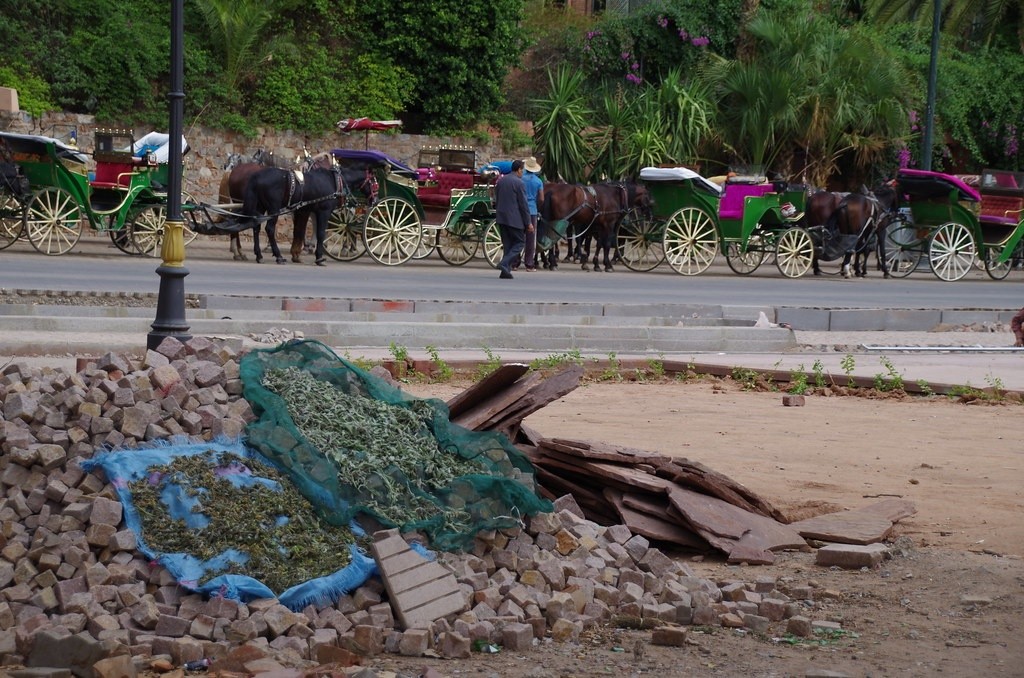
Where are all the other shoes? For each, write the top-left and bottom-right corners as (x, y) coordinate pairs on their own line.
(526, 267), (537, 272)
(511, 265), (519, 271)
(497, 260), (514, 279)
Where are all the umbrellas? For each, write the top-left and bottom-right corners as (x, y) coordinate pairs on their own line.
(336, 117), (403, 152)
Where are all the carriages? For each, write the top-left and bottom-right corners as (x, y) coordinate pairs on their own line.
(614, 165), (902, 279)
(330, 116), (654, 273)
(0, 106), (380, 266)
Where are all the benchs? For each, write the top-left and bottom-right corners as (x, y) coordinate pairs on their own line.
(718, 183), (773, 220)
(977, 193), (1023, 225)
(415, 171), (474, 209)
(90, 162), (141, 201)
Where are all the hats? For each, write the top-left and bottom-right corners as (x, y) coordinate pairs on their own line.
(522, 157), (541, 172)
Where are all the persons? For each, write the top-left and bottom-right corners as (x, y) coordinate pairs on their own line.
(511, 156), (544, 272)
(495, 160), (534, 279)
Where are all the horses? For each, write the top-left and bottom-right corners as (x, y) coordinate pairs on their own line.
(522, 175), (652, 273)
(804, 184), (906, 281)
(214, 151), (380, 267)
